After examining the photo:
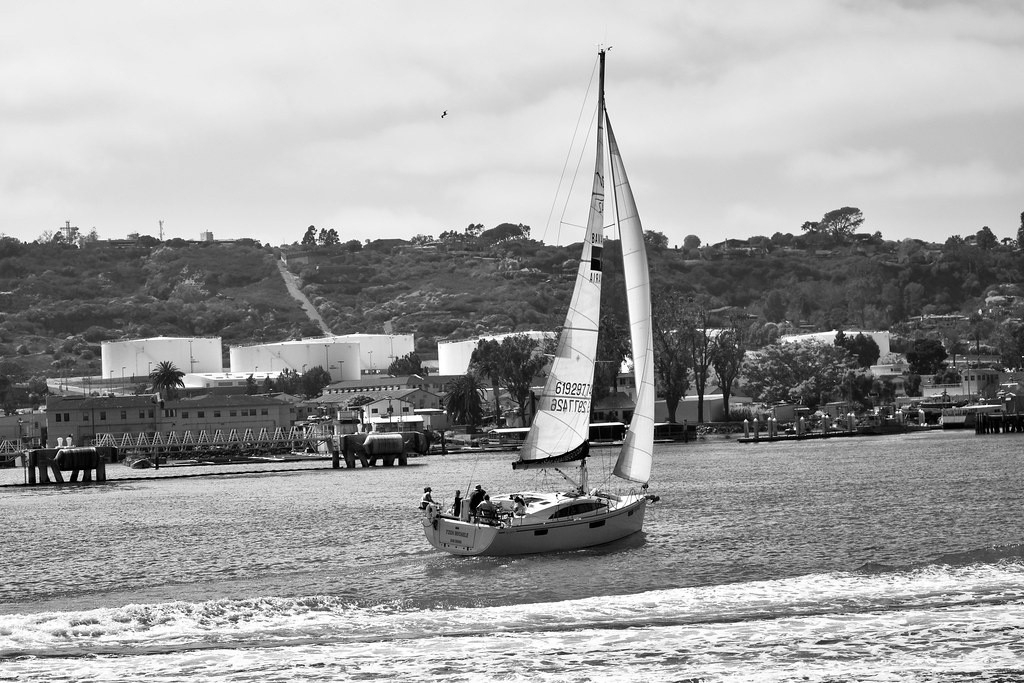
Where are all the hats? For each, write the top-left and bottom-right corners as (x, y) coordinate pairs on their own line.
(423, 487), (432, 492)
(474, 484), (481, 490)
(483, 494), (490, 499)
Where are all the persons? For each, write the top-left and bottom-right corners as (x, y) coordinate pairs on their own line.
(514, 497), (525, 516)
(420, 487), (438, 511)
(470, 485), (486, 522)
(454, 490), (463, 517)
(477, 495), (498, 523)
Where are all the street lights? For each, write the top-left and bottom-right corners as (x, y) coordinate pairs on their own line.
(325, 344), (330, 373)
(83, 371), (86, 397)
(966, 332), (973, 406)
(368, 350), (373, 376)
(148, 362), (152, 380)
(64, 371), (69, 392)
(59, 364), (63, 393)
(188, 340), (200, 373)
(88, 371), (90, 396)
(417, 41), (661, 557)
(16, 417), (24, 452)
(122, 367), (126, 396)
(110, 370), (114, 395)
(388, 336), (398, 363)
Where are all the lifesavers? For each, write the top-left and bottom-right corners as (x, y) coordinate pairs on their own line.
(424, 503), (437, 521)
